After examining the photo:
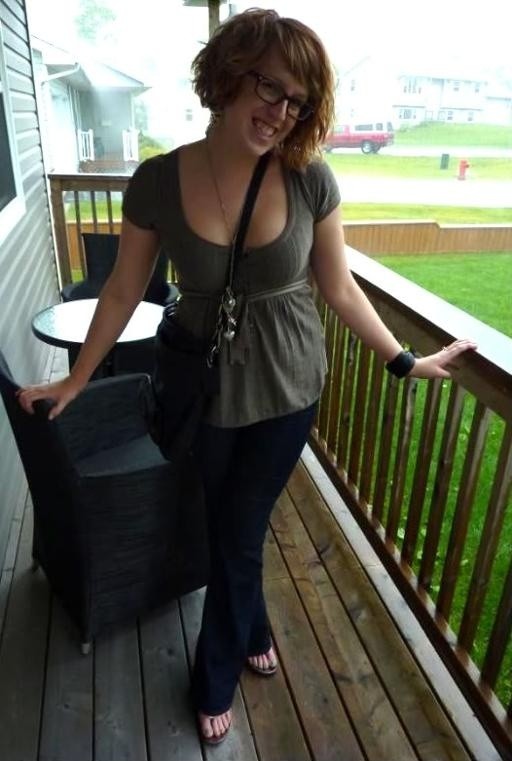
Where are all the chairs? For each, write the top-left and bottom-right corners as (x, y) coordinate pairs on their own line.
(61, 232), (178, 304)
(0, 353), (208, 657)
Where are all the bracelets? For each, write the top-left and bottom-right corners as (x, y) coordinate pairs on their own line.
(384, 349), (416, 379)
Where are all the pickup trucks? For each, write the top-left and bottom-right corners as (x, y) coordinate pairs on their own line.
(320, 124), (394, 154)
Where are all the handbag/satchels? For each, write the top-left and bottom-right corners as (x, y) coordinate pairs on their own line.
(137, 298), (218, 463)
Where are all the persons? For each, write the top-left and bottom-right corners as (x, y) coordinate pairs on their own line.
(13, 6), (477, 745)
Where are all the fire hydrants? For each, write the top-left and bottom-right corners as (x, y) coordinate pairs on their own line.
(458, 159), (469, 180)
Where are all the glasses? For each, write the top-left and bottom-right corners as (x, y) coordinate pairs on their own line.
(248, 69), (318, 122)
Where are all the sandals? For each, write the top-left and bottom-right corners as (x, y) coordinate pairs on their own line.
(246, 646), (279, 677)
(196, 707), (234, 746)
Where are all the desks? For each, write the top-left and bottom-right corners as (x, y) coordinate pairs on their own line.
(31, 297), (165, 382)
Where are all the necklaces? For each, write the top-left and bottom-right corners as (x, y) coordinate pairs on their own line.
(203, 137), (244, 242)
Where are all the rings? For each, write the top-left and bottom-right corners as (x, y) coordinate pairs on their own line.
(441, 345), (449, 351)
